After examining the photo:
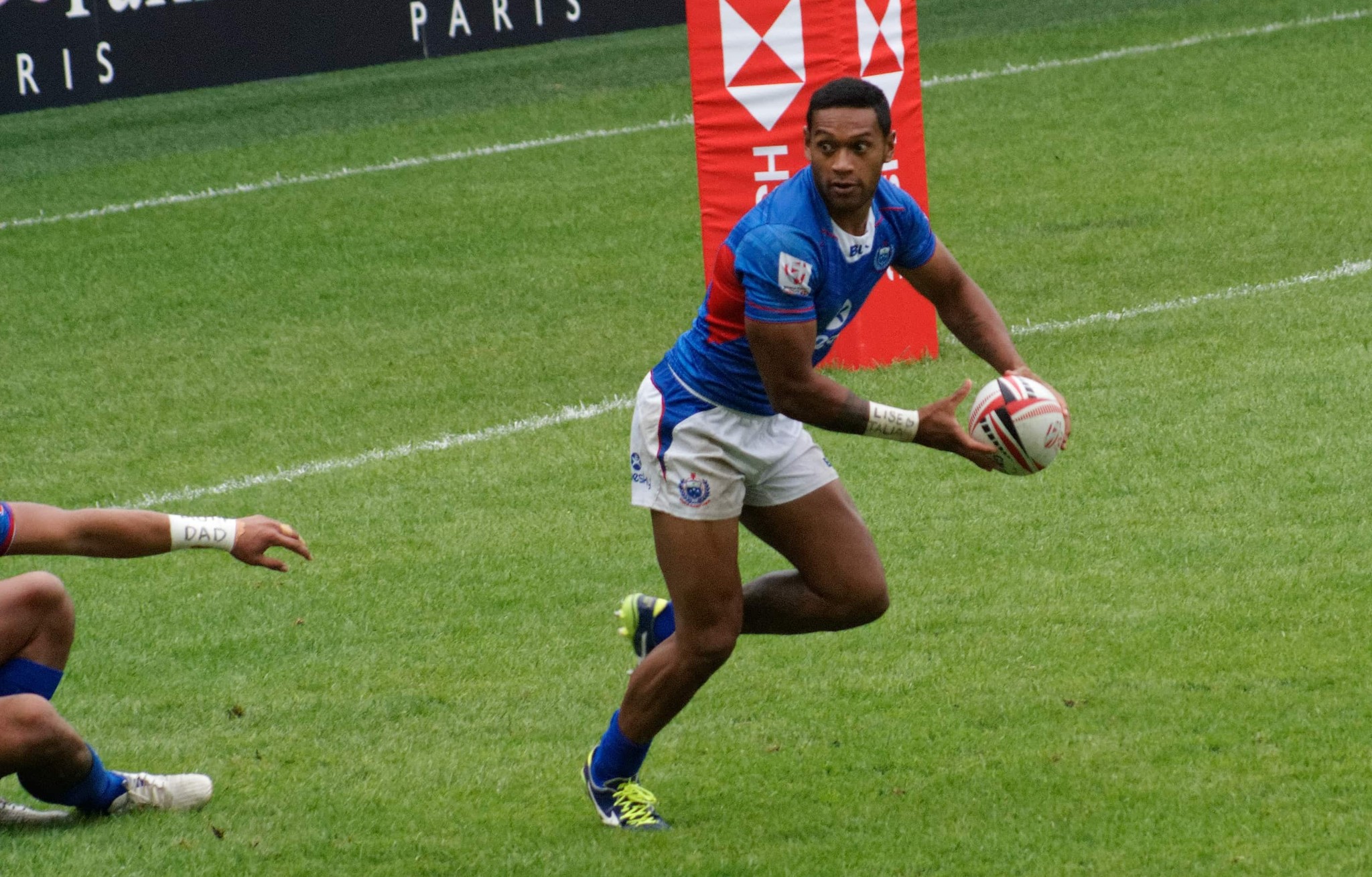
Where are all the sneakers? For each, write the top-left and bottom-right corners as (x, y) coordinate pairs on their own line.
(613, 593), (670, 675)
(78, 772), (212, 819)
(0, 798), (72, 829)
(580, 744), (673, 834)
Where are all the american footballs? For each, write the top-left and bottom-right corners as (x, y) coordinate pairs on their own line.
(968, 375), (1066, 476)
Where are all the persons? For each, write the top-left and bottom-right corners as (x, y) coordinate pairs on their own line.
(580, 76), (1070, 830)
(0, 501), (313, 827)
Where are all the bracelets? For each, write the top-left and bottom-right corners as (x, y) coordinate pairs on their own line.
(862, 402), (921, 444)
(169, 513), (236, 555)
